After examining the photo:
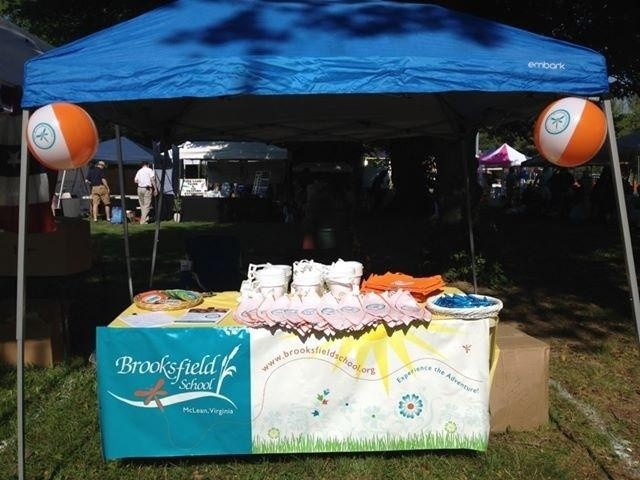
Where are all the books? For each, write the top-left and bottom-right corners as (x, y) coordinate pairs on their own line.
(174, 307), (231, 324)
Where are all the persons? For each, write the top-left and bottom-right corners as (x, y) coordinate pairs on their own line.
(87, 161), (111, 221)
(134, 161), (158, 225)
(504, 168), (516, 207)
(371, 171), (388, 202)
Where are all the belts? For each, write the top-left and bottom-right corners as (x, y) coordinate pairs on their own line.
(138, 186), (152, 188)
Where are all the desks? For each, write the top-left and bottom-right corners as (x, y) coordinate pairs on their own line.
(97, 287), (491, 453)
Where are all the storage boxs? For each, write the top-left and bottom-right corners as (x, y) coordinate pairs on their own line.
(491, 323), (552, 433)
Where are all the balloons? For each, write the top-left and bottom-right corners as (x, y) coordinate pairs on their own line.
(26, 102), (98, 170)
(533, 97), (607, 168)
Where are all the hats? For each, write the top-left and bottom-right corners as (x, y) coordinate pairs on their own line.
(98, 161), (105, 165)
